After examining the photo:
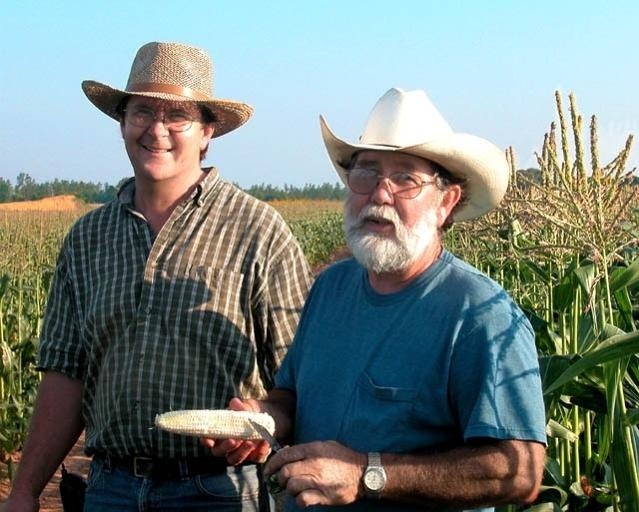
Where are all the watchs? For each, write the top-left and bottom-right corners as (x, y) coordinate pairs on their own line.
(363, 451), (386, 502)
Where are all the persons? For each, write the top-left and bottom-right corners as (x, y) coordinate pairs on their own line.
(193, 82), (550, 511)
(1, 37), (313, 512)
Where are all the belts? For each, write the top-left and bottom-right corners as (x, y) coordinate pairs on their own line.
(94, 453), (227, 478)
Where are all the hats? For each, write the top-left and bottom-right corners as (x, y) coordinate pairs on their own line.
(319, 88), (509, 222)
(82, 42), (253, 138)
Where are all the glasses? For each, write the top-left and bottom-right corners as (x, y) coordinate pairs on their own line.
(124, 106), (198, 132)
(346, 169), (436, 198)
